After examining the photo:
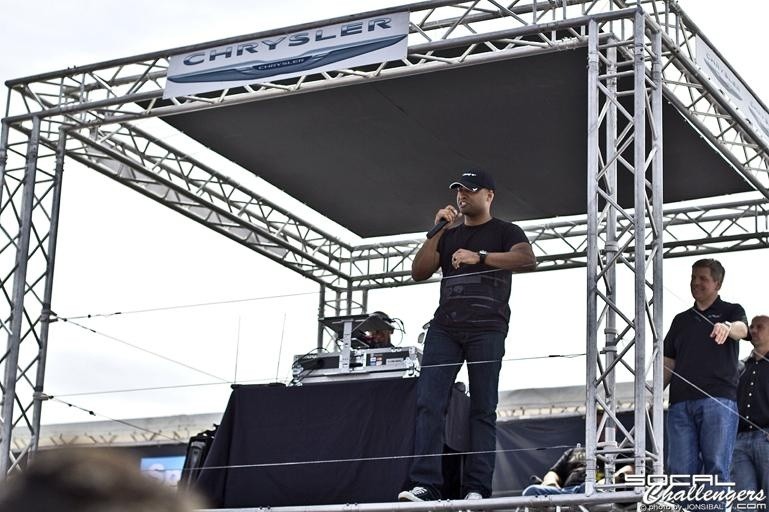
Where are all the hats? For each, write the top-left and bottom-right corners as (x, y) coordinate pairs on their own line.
(449, 166), (496, 193)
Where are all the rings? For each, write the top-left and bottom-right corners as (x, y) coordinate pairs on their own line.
(454, 257), (458, 262)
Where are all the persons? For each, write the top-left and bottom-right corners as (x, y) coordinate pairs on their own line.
(396, 167), (538, 501)
(730, 315), (769, 512)
(1, 442), (204, 510)
(522, 411), (639, 508)
(367, 311), (394, 349)
(664, 256), (751, 512)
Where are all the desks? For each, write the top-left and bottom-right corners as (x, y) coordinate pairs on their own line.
(232, 376), (472, 509)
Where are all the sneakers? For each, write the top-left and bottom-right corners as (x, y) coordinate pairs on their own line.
(397, 483), (442, 502)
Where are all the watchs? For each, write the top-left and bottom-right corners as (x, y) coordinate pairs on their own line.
(478, 249), (488, 264)
(722, 320), (733, 331)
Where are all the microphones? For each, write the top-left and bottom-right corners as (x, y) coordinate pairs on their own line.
(426, 206), (460, 239)
